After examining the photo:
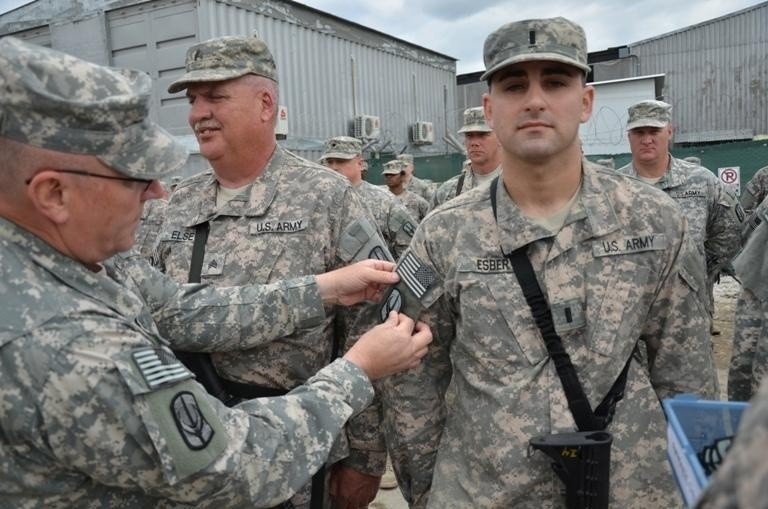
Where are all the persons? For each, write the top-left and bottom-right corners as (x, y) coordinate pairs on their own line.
(0, 35), (431, 509)
(129, 18), (767, 509)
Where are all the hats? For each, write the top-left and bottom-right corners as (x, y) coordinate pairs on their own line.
(381, 160), (406, 174)
(457, 106), (492, 134)
(396, 154), (415, 164)
(168, 36), (279, 92)
(1, 36), (190, 181)
(319, 137), (361, 160)
(480, 16), (592, 78)
(625, 101), (672, 131)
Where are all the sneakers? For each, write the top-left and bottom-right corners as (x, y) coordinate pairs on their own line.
(380, 455), (398, 488)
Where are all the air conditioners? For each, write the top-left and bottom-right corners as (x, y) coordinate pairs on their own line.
(273, 104), (288, 139)
(413, 120), (434, 143)
(354, 114), (380, 140)
